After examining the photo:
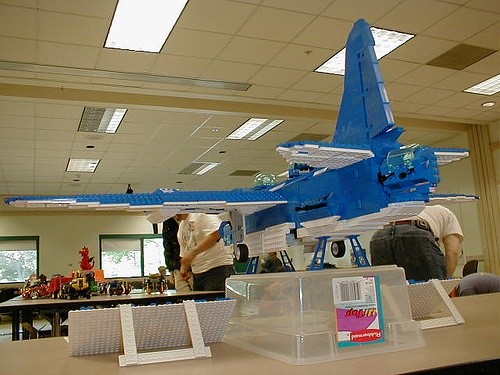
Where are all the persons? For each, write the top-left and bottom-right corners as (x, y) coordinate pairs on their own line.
(259, 252), (282, 274)
(162, 212), (237, 291)
(449, 272), (500, 298)
(370, 203), (463, 283)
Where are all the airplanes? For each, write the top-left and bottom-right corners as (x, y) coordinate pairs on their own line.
(4, 16), (482, 271)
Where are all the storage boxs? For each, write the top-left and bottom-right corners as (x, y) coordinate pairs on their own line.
(225, 264), (426, 366)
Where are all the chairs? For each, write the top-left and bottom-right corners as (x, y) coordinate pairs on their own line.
(463, 260), (478, 277)
(456, 272), (500, 297)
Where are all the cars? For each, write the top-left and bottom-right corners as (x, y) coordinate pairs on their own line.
(17, 271), (169, 298)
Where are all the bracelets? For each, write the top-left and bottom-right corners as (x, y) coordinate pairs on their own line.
(191, 245), (201, 255)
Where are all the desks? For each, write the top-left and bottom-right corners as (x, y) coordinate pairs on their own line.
(0, 289), (500, 375)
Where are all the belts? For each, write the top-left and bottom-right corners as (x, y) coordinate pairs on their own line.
(383, 220), (434, 237)
